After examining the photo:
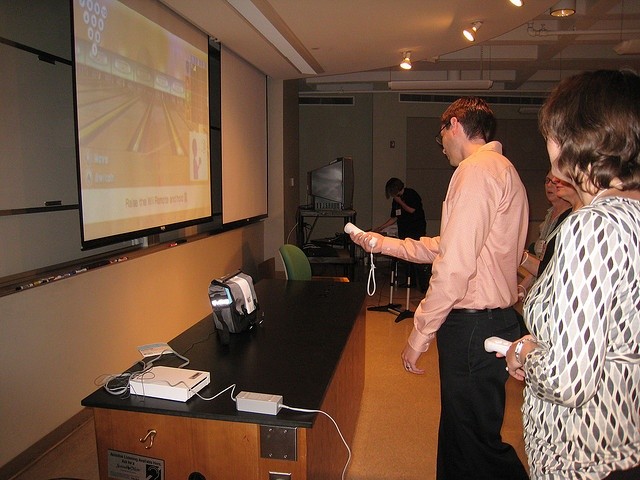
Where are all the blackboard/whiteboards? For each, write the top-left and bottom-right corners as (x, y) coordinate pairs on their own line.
(0, 0), (221, 298)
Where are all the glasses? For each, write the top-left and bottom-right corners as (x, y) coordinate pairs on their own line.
(435, 122), (450, 146)
(544, 176), (552, 184)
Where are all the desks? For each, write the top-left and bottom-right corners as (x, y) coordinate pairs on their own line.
(81, 275), (370, 480)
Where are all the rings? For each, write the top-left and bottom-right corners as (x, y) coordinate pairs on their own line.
(406, 365), (410, 369)
(505, 367), (509, 371)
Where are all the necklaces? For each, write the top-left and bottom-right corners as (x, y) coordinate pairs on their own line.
(589, 181), (623, 206)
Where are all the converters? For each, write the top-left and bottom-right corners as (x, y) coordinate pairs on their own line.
(236, 390), (283, 417)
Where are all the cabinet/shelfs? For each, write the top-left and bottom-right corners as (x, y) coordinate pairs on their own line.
(297, 206), (357, 269)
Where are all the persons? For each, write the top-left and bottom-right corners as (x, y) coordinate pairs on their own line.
(350, 96), (528, 479)
(528, 169), (570, 258)
(370, 178), (427, 288)
(495, 67), (640, 478)
(516, 165), (584, 278)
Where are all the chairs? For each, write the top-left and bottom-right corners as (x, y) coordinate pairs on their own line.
(279, 244), (311, 280)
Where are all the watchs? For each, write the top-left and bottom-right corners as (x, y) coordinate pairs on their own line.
(514, 338), (528, 366)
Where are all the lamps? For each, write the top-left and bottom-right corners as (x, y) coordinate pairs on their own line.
(612, 0), (640, 57)
(399, 51), (412, 69)
(517, 87), (540, 115)
(463, 21), (483, 42)
(510, 0), (526, 7)
(387, 42), (494, 90)
(550, 0), (576, 18)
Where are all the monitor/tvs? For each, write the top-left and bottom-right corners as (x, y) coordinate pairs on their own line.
(306, 156), (355, 211)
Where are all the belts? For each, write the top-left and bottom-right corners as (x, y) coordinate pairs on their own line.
(450, 308), (499, 314)
